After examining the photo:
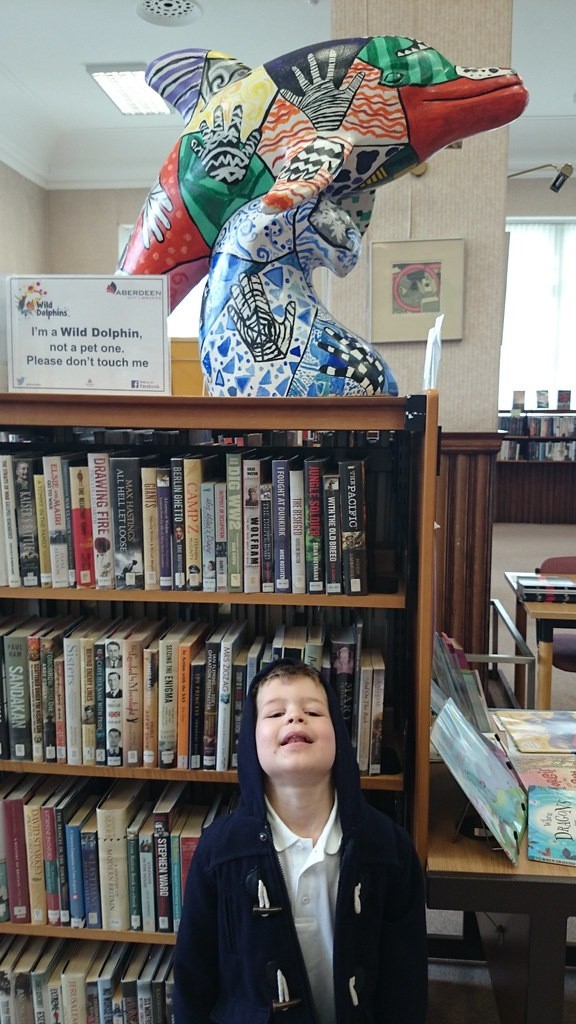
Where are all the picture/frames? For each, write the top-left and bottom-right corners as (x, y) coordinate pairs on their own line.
(368, 237), (464, 343)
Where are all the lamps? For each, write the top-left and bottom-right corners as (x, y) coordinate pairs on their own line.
(89, 64), (175, 116)
(508, 162), (574, 193)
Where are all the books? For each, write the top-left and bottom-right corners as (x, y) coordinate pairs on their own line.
(0, 430), (415, 1023)
(515, 575), (576, 603)
(430, 630), (576, 866)
(497, 389), (576, 461)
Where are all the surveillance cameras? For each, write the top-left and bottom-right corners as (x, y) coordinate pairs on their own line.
(548, 163), (574, 193)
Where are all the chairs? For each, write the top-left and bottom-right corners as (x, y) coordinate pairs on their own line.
(535, 555), (576, 673)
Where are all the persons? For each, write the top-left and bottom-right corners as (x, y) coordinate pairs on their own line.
(171, 658), (430, 1024)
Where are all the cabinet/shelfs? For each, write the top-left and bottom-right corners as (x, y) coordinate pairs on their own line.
(493, 410), (576, 526)
(0, 380), (436, 947)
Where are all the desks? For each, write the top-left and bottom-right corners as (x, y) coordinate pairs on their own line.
(422, 759), (576, 1023)
(505, 571), (576, 711)
